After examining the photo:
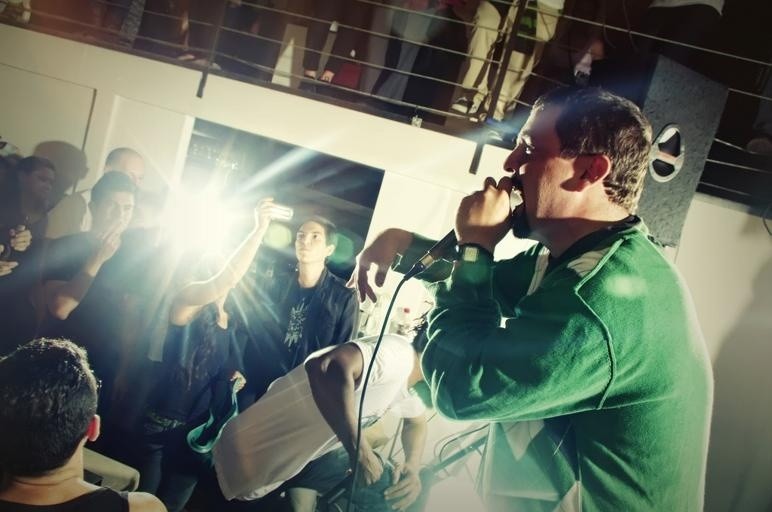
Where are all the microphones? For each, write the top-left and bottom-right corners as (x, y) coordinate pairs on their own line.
(404, 187), (525, 281)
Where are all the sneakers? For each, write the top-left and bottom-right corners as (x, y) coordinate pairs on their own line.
(452, 97), (478, 114)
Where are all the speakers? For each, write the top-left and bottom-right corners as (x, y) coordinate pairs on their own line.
(605, 46), (731, 249)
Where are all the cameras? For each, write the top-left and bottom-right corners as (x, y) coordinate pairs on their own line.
(271, 204), (294, 221)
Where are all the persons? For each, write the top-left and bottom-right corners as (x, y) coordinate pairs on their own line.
(340, 84), (714, 512)
